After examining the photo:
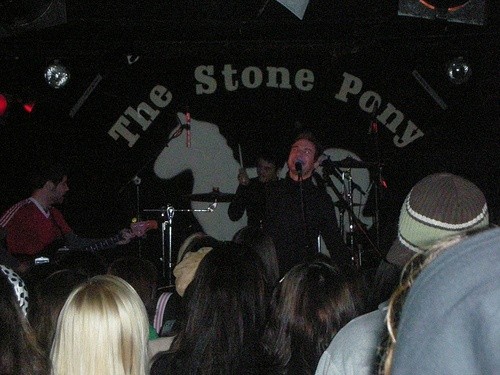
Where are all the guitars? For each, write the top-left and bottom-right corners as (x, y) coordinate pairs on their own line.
(15, 219), (158, 290)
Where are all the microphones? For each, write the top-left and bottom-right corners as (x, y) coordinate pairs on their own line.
(295, 162), (303, 175)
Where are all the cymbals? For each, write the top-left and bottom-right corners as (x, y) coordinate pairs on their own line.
(327, 157), (374, 169)
(181, 187), (235, 203)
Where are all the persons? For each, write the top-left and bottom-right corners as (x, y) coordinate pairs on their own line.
(250, 130), (349, 263)
(0, 130), (500, 375)
(49, 273), (152, 375)
(378, 226), (500, 375)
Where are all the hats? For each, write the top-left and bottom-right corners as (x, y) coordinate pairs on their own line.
(385, 172), (490, 269)
